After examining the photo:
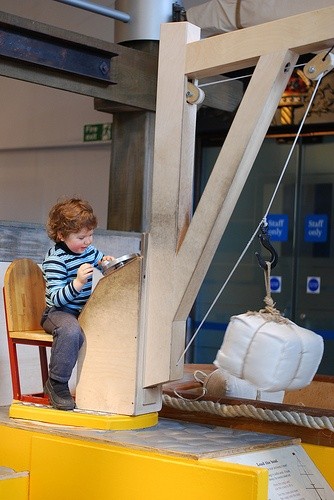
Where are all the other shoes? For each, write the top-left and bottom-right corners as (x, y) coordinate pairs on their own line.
(43, 378), (75, 410)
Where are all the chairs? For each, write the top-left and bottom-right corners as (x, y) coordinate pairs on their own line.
(2, 258), (76, 406)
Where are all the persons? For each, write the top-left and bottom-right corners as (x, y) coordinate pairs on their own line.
(40, 199), (119, 412)
